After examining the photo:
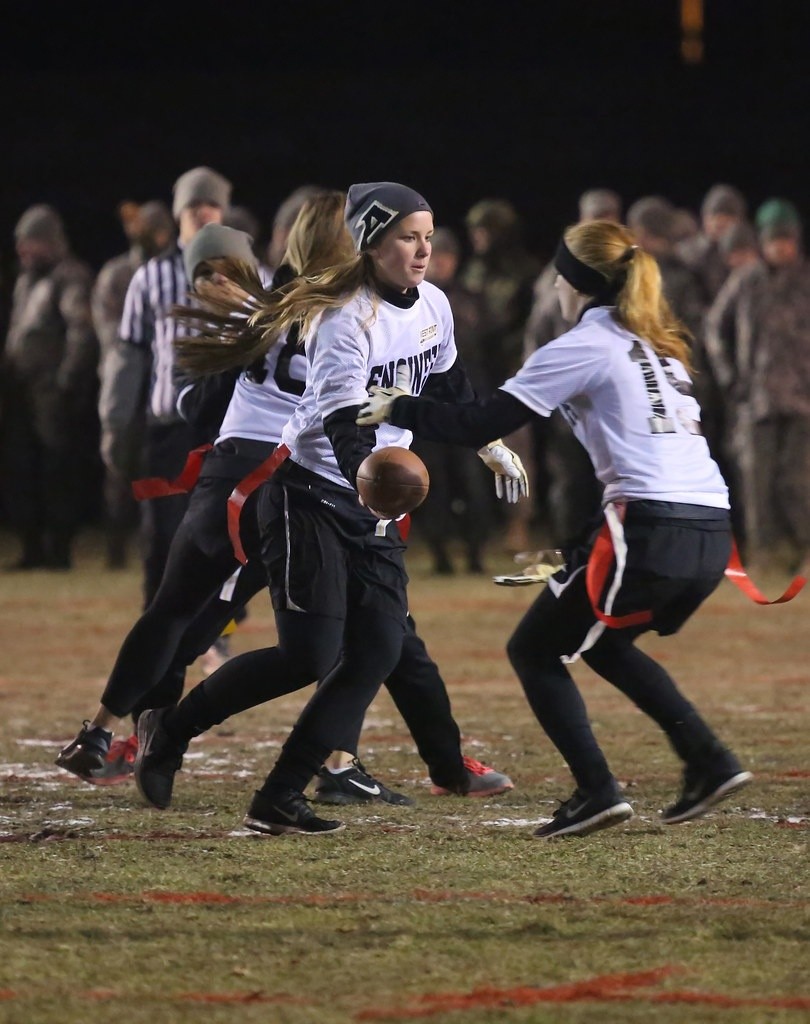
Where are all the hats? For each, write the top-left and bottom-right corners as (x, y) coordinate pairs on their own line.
(172, 165), (234, 213)
(629, 197), (674, 234)
(184, 222), (260, 282)
(344, 181), (434, 250)
(700, 182), (748, 217)
(754, 196), (798, 232)
(464, 199), (511, 228)
(427, 223), (460, 256)
(14, 204), (66, 243)
(720, 225), (753, 257)
(579, 187), (623, 219)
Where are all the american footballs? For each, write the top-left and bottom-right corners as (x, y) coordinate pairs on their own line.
(356, 446), (430, 515)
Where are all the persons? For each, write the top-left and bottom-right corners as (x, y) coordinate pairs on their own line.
(134, 184), (529, 839)
(356, 221), (755, 838)
(55, 182), (416, 810)
(82, 220), (516, 796)
(100, 168), (248, 675)
(0, 207), (88, 578)
(86, 186), (810, 574)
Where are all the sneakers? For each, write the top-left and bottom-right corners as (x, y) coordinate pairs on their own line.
(77, 732), (150, 785)
(315, 759), (417, 807)
(432, 754), (516, 798)
(55, 722), (115, 769)
(530, 775), (635, 840)
(241, 787), (346, 837)
(132, 705), (189, 810)
(10, 526), (810, 591)
(656, 750), (756, 821)
